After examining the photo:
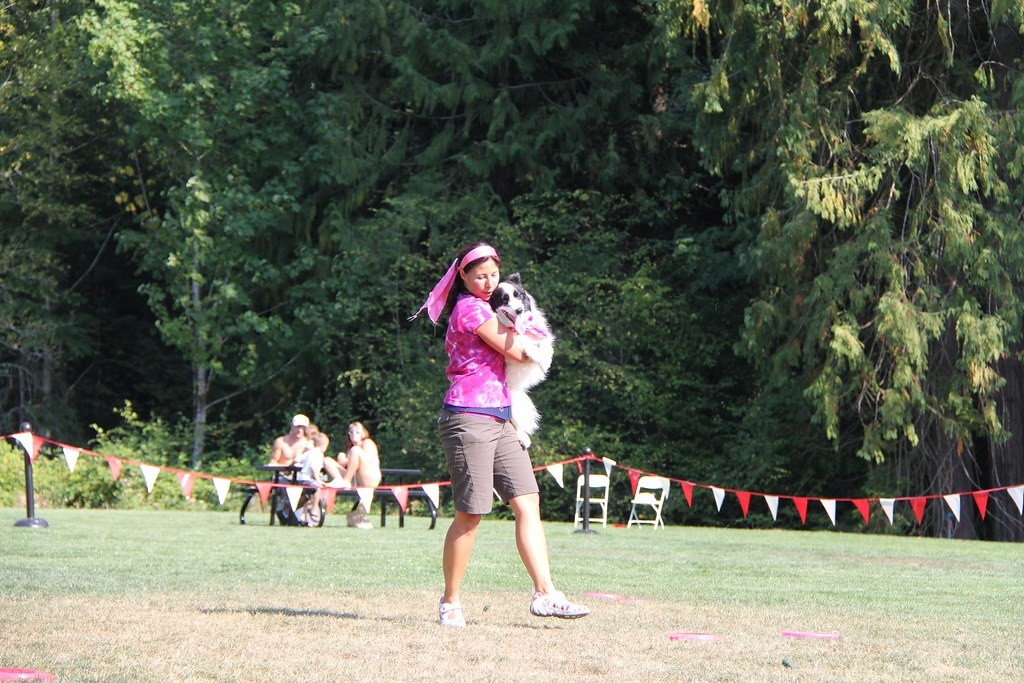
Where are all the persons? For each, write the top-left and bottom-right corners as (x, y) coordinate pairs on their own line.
(297, 433), (330, 521)
(270, 414), (319, 466)
(323, 423), (382, 492)
(437, 243), (589, 629)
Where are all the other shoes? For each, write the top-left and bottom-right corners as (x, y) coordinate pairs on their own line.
(344, 482), (352, 489)
(325, 479), (345, 488)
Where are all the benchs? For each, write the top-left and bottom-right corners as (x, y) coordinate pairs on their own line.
(305, 487), (444, 499)
(238, 487), (261, 493)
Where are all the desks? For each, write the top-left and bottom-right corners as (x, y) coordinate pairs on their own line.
(237, 466), (437, 531)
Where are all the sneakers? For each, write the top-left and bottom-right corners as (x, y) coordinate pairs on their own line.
(531, 592), (591, 618)
(438, 597), (465, 631)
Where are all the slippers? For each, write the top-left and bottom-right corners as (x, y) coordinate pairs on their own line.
(308, 508), (320, 522)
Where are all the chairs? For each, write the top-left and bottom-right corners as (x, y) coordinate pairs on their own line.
(627, 476), (669, 530)
(574, 474), (609, 528)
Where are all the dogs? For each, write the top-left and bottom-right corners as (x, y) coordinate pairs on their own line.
(489, 271), (557, 449)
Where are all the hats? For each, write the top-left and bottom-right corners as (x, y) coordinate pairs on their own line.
(292, 414), (309, 427)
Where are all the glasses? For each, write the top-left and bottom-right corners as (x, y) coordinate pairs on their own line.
(347, 430), (361, 433)
